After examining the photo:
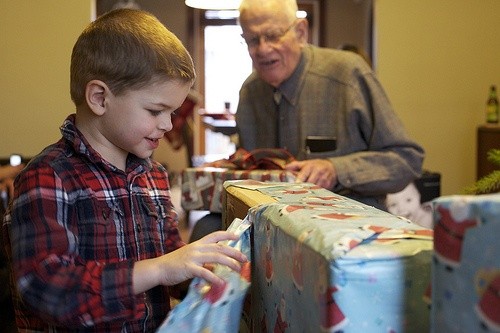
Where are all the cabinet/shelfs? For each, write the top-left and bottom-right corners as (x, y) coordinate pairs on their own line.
(475, 124), (500, 194)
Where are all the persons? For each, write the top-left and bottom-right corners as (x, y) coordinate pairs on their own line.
(189, 0), (426, 244)
(164, 86), (196, 171)
(1, 7), (246, 333)
(382, 182), (428, 226)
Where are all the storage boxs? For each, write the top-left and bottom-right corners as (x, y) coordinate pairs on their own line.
(180, 167), (302, 212)
(222, 180), (434, 333)
(433, 193), (500, 333)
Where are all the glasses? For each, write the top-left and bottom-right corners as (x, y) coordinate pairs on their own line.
(248, 20), (297, 45)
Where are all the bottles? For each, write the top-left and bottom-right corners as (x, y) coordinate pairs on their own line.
(486, 86), (500, 124)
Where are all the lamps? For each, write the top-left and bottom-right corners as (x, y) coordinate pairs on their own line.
(184, 1), (242, 10)
